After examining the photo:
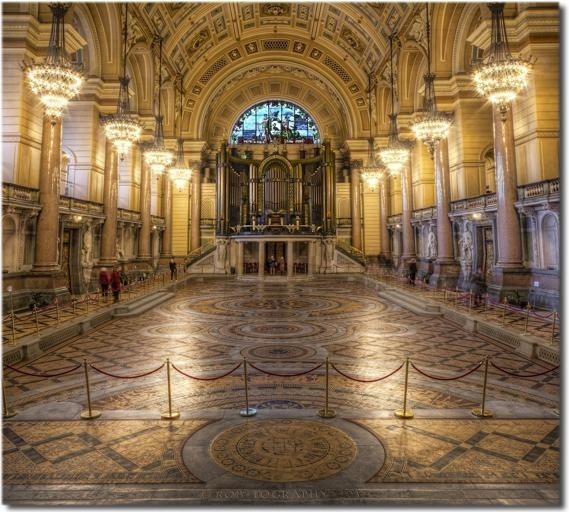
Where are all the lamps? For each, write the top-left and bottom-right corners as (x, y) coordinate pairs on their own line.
(356, 3), (450, 192)
(472, 3), (537, 124)
(22, 2), (87, 129)
(100, 1), (193, 192)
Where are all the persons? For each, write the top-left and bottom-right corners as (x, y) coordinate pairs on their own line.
(98, 267), (111, 297)
(406, 258), (417, 284)
(279, 257), (284, 276)
(109, 269), (123, 304)
(458, 225), (472, 260)
(268, 255), (277, 276)
(469, 265), (484, 305)
(423, 260), (433, 285)
(169, 257), (178, 282)
(425, 227), (436, 256)
